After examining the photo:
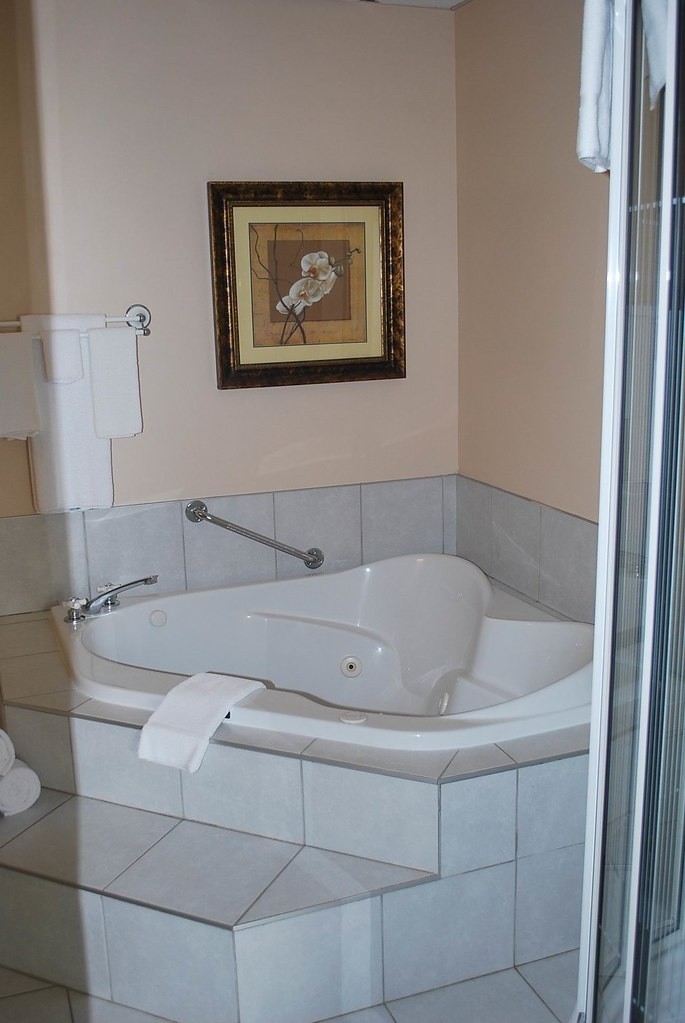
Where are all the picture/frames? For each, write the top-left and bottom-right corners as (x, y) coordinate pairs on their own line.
(207, 180), (407, 390)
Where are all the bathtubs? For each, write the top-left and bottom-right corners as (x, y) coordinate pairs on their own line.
(50, 553), (638, 753)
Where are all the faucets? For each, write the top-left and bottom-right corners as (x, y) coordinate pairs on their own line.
(85, 575), (159, 613)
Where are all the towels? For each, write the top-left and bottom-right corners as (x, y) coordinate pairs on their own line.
(0, 729), (16, 777)
(39, 330), (83, 385)
(18, 314), (114, 515)
(0, 758), (41, 817)
(576, 0), (668, 174)
(87, 327), (144, 438)
(137, 671), (267, 773)
(0, 332), (43, 440)
